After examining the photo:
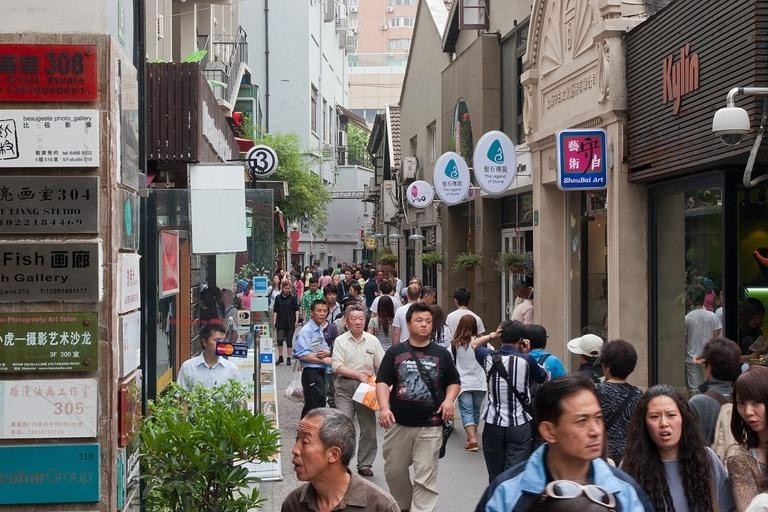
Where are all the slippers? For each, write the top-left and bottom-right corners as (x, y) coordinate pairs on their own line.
(464, 442), (479, 451)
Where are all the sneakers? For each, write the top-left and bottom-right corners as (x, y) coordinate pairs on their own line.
(286, 357), (291, 365)
(358, 469), (373, 478)
(276, 356), (283, 366)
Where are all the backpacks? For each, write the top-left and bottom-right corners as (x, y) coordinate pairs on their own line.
(702, 390), (748, 475)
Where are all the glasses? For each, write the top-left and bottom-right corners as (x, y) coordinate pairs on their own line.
(546, 479), (616, 508)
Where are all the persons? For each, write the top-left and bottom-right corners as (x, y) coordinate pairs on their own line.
(365, 262), (377, 272)
(268, 273), (281, 328)
(348, 284), (365, 305)
(280, 407), (402, 512)
(375, 303), (461, 512)
(329, 262), (363, 269)
(392, 284), (421, 346)
(355, 270), (366, 289)
(445, 287), (486, 338)
(280, 270), (284, 276)
(292, 299), (332, 421)
(323, 283), (341, 323)
(273, 280), (299, 366)
(335, 295), (355, 320)
(739, 297), (767, 360)
(686, 279), (725, 386)
(242, 281), (254, 309)
(313, 271), (325, 291)
(727, 366), (768, 511)
(328, 301), (361, 345)
(365, 272), (378, 306)
(471, 319), (549, 485)
(304, 263), (318, 272)
(332, 305), (386, 477)
(369, 296), (395, 350)
(428, 305), (453, 348)
(318, 269), (331, 293)
(511, 280), (534, 324)
(388, 269), (402, 292)
(293, 273), (305, 328)
(566, 334), (604, 389)
(176, 322), (246, 415)
(330, 274), (339, 287)
(301, 278), (325, 323)
(447, 315), (487, 452)
(403, 278), (422, 296)
(689, 336), (742, 447)
(369, 282), (403, 317)
(521, 324), (567, 383)
(475, 375), (655, 512)
(376, 271), (388, 288)
(235, 271), (258, 306)
(218, 288), (238, 321)
(619, 385), (731, 512)
(590, 340), (645, 469)
(339, 269), (359, 296)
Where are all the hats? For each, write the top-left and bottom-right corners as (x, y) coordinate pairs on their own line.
(566, 333), (603, 357)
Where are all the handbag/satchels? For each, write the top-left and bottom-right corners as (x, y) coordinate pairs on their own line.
(404, 340), (453, 459)
